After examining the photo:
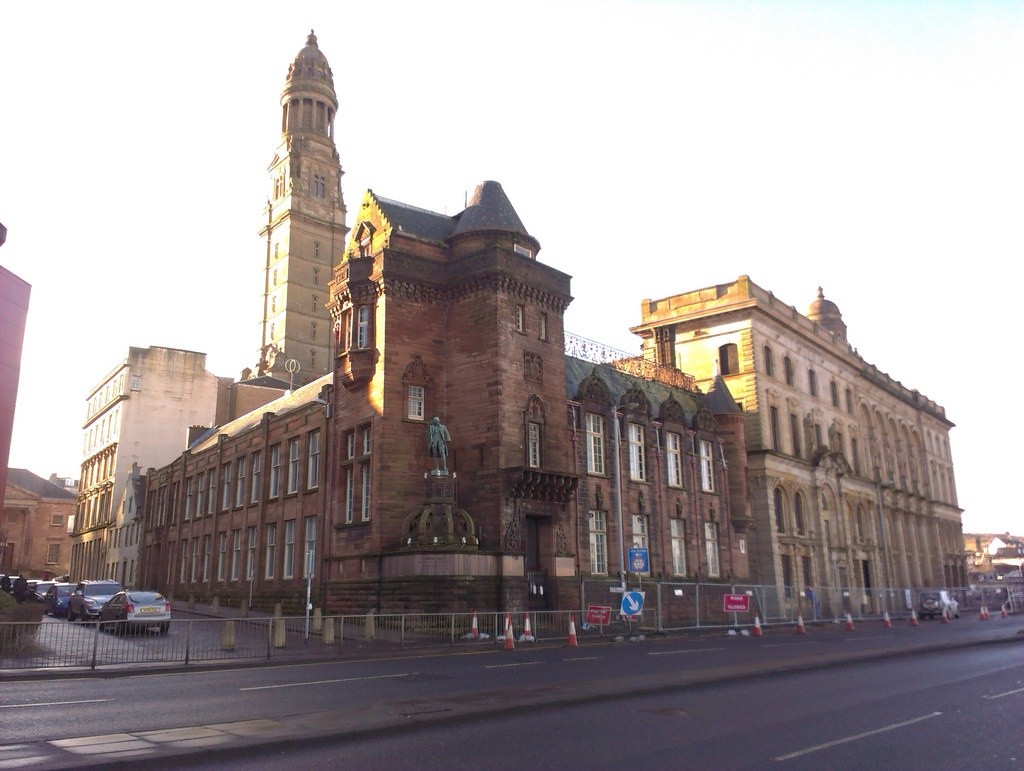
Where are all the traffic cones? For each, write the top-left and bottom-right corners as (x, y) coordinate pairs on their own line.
(910, 607), (921, 626)
(563, 615), (581, 648)
(845, 610), (857, 631)
(520, 612), (535, 643)
(504, 616), (520, 650)
(469, 609), (482, 641)
(500, 610), (511, 640)
(1000, 604), (1009, 619)
(751, 611), (764, 638)
(984, 605), (990, 619)
(942, 609), (950, 624)
(882, 608), (894, 629)
(980, 606), (987, 620)
(793, 612), (814, 635)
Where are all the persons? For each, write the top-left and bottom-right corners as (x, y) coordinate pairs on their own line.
(428, 417), (451, 471)
(0, 572), (11, 592)
(12, 575), (28, 603)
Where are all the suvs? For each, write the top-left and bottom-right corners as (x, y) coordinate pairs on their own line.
(68, 579), (126, 626)
(917, 589), (962, 621)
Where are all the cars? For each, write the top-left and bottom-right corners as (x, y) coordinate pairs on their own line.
(29, 581), (60, 610)
(7, 575), (20, 595)
(24, 579), (46, 602)
(97, 590), (172, 635)
(46, 583), (81, 618)
(0, 574), (7, 588)
